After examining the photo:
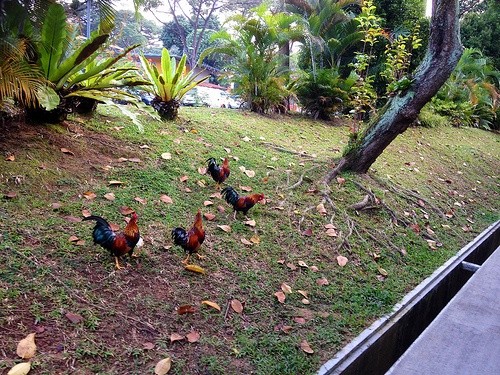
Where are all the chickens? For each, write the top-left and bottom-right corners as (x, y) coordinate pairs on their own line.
(170, 208), (207, 266)
(80, 211), (140, 272)
(202, 157), (231, 191)
(220, 186), (265, 222)
